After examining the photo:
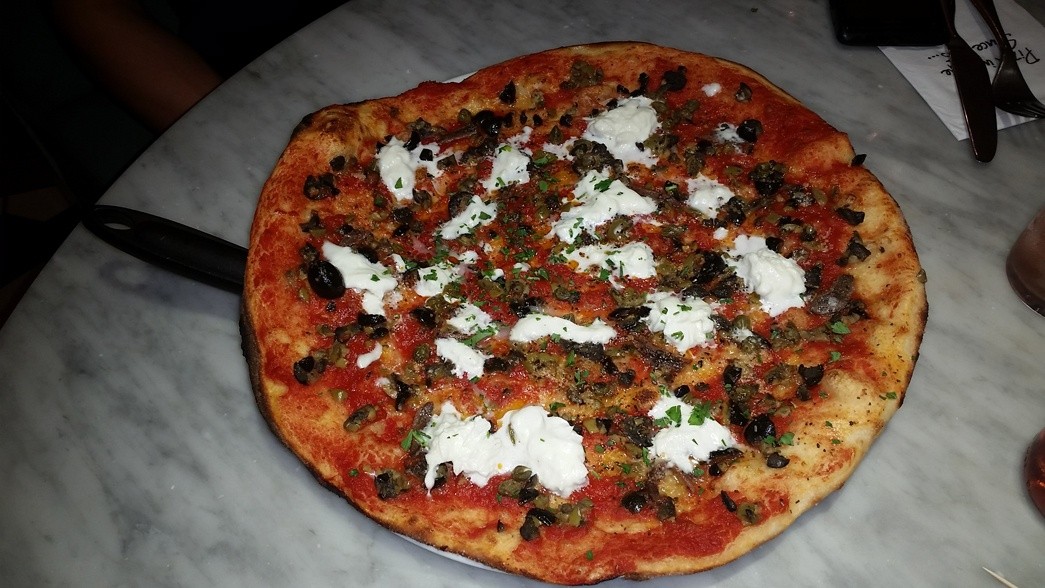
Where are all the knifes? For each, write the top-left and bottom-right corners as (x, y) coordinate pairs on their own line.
(936, 0), (998, 164)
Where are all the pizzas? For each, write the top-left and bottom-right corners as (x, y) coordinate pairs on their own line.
(245, 42), (927, 584)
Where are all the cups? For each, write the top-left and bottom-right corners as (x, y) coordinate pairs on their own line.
(1024, 428), (1045, 520)
(1006, 203), (1045, 319)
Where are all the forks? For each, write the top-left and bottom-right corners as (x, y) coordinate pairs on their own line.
(968, 0), (1045, 119)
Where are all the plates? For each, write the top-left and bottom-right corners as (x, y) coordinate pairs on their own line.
(393, 71), (521, 576)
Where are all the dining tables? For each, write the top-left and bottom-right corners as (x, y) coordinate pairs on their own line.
(0, 0), (1045, 588)
